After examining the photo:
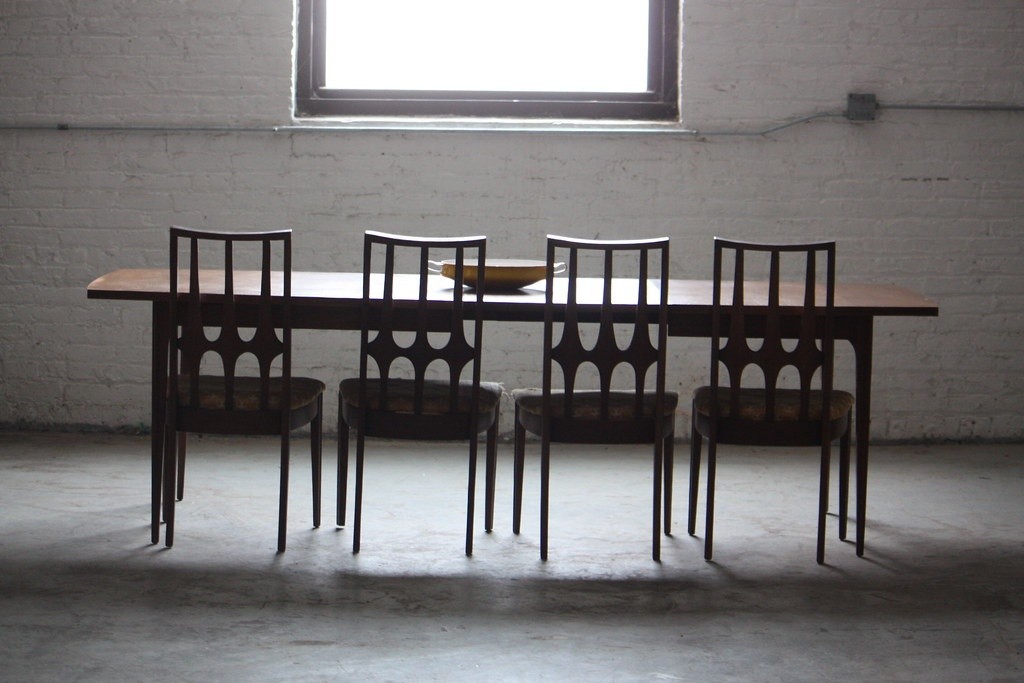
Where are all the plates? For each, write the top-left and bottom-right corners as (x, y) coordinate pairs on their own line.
(428, 258), (567, 291)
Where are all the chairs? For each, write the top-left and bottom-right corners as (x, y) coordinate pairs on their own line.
(336, 230), (506, 554)
(688, 235), (854, 565)
(511, 234), (679, 561)
(165, 225), (326, 553)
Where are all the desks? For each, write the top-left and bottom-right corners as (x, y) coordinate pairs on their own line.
(85, 268), (942, 555)
